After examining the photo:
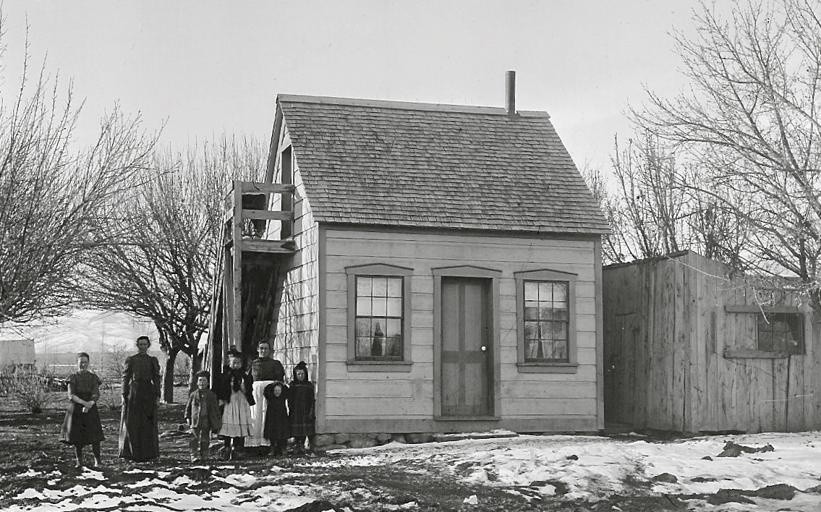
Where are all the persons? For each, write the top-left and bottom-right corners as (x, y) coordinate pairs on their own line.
(57, 351), (106, 469)
(183, 340), (316, 463)
(370, 322), (384, 356)
(118, 335), (161, 464)
